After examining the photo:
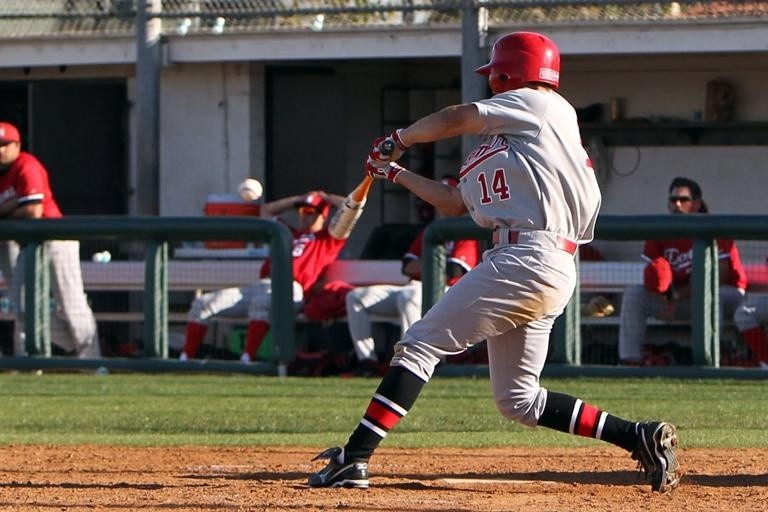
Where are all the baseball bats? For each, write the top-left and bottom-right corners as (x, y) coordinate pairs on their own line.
(327, 141), (394, 240)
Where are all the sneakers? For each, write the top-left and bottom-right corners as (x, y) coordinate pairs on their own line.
(631, 421), (683, 496)
(339, 359), (392, 377)
(308, 447), (369, 489)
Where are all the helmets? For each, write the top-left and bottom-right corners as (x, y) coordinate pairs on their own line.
(473, 31), (560, 94)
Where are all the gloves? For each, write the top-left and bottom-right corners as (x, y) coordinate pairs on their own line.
(361, 128), (412, 183)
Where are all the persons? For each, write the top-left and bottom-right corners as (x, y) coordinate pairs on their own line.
(0, 121), (111, 377)
(304, 30), (686, 493)
(734, 293), (768, 370)
(616, 173), (748, 364)
(336, 176), (483, 379)
(548, 244), (617, 362)
(165, 190), (349, 367)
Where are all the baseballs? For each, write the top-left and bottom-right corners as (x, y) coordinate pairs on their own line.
(237, 178), (263, 201)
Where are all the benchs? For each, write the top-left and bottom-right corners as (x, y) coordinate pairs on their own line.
(80, 260), (694, 364)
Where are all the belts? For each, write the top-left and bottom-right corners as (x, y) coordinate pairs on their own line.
(492, 230), (577, 255)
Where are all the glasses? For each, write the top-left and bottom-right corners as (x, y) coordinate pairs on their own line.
(668, 196), (691, 203)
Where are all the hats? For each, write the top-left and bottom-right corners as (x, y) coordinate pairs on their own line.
(644, 256), (673, 294)
(0, 122), (21, 142)
(293, 191), (332, 220)
